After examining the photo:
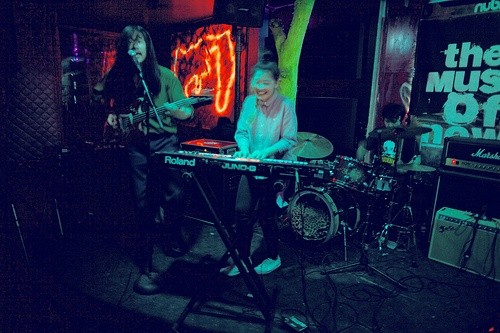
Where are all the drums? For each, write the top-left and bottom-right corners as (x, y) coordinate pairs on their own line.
(332, 153), (375, 193)
(368, 174), (393, 195)
(287, 184), (360, 245)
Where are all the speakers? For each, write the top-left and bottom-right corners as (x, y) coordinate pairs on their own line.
(427, 207), (500, 282)
(212, 0), (265, 28)
(425, 169), (500, 245)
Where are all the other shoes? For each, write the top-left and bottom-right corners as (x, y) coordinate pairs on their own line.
(386, 240), (397, 250)
(219, 256), (252, 277)
(138, 254), (165, 294)
(254, 254), (282, 275)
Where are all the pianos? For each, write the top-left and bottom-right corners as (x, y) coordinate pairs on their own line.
(153, 150), (337, 330)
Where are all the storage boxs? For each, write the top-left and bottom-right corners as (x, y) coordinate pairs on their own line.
(180, 139), (238, 156)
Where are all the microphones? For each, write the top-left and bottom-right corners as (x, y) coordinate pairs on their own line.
(128, 49), (137, 56)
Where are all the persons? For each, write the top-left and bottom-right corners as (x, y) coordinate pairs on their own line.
(220, 52), (298, 276)
(366, 103), (419, 239)
(102, 25), (195, 275)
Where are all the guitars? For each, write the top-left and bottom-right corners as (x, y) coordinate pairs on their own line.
(100, 93), (214, 159)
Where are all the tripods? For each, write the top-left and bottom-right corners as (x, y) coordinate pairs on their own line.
(318, 135), (423, 292)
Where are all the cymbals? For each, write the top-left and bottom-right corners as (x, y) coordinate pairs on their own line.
(396, 161), (437, 174)
(368, 126), (433, 139)
(295, 130), (334, 160)
